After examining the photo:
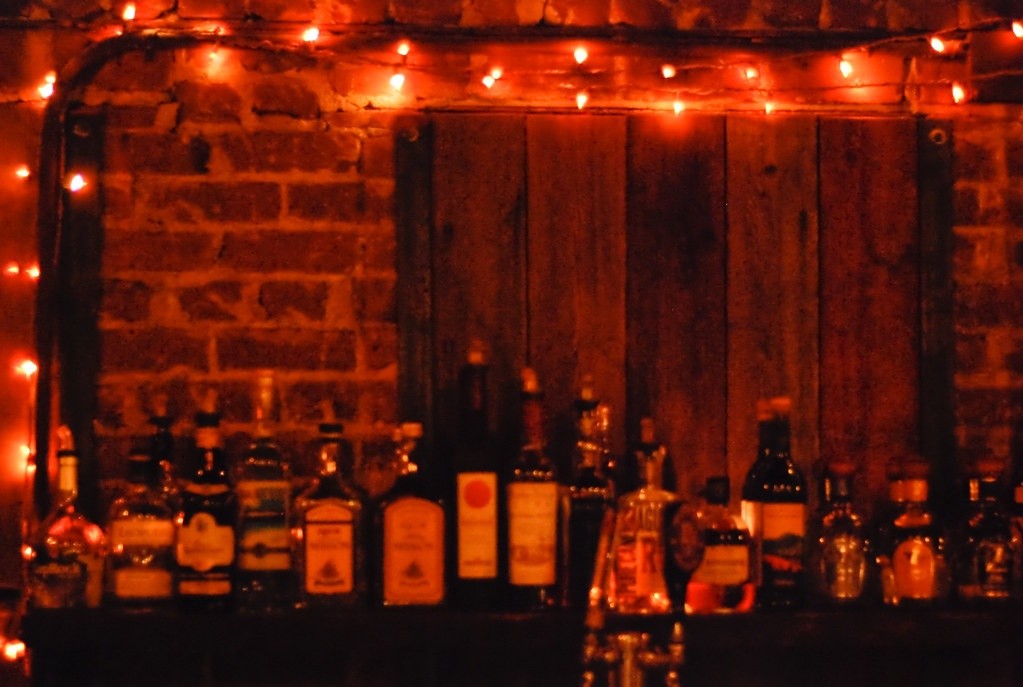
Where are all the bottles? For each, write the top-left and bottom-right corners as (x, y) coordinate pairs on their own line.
(0, 392), (371, 687)
(370, 391), (1023, 687)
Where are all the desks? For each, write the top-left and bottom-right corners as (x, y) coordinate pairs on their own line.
(20, 605), (1023, 687)
